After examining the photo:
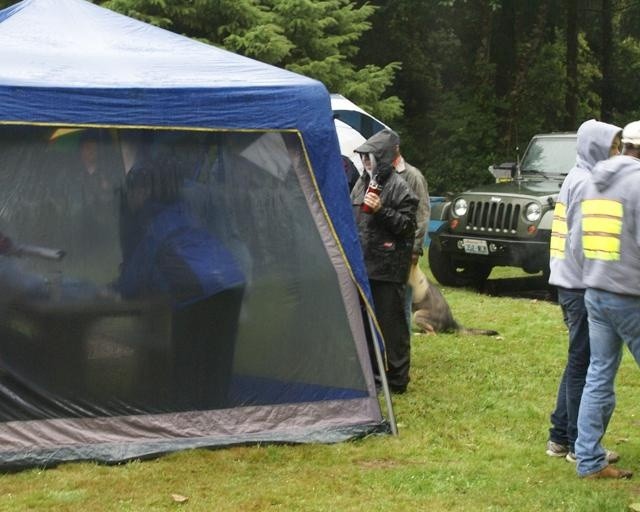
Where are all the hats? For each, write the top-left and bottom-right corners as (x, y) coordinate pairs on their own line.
(620, 120), (640, 146)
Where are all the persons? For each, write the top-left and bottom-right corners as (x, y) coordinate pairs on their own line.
(349, 129), (419, 398)
(374, 128), (429, 363)
(575, 118), (639, 481)
(117, 192), (248, 408)
(543, 118), (624, 469)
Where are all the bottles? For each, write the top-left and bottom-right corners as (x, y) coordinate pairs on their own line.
(360, 179), (383, 215)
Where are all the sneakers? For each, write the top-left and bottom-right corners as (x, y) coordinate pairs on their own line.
(579, 463), (634, 480)
(566, 448), (620, 465)
(546, 440), (569, 457)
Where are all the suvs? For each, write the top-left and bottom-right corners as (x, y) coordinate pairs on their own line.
(422, 196), (453, 245)
(428, 132), (581, 286)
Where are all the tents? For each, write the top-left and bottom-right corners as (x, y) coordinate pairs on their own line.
(2, 0), (401, 471)
